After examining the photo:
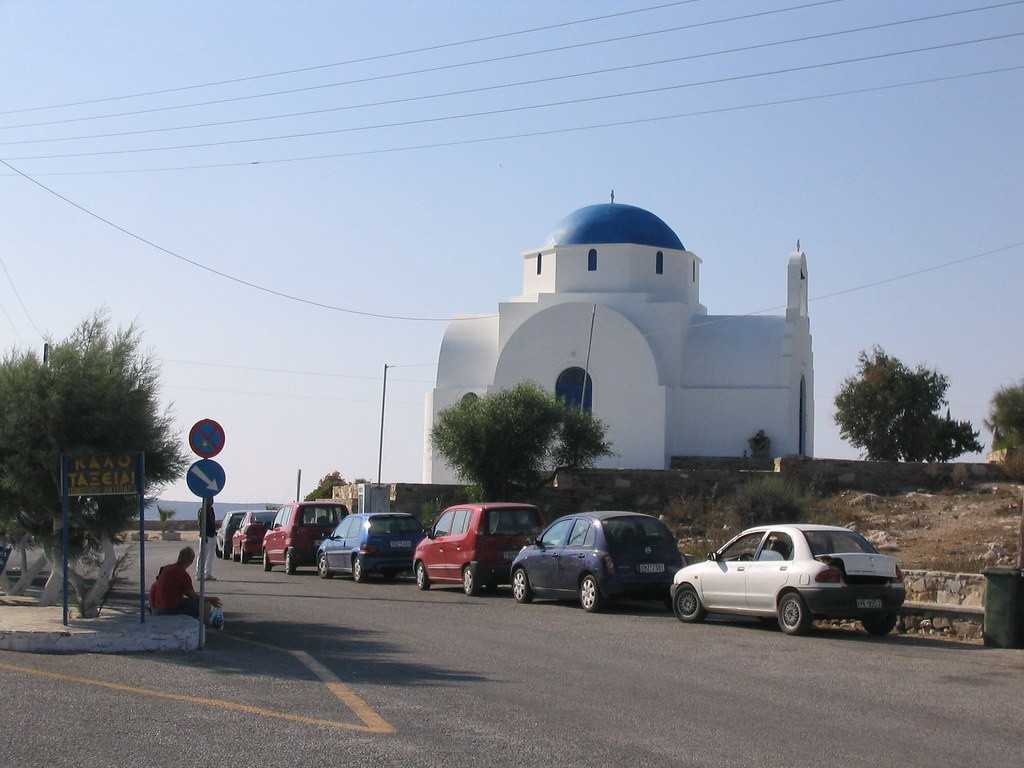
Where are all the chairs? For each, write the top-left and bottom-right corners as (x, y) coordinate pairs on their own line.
(770, 541), (789, 560)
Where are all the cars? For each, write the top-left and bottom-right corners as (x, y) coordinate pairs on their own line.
(510, 511), (690, 612)
(231, 510), (278, 564)
(262, 501), (350, 575)
(412, 502), (548, 596)
(215, 510), (248, 559)
(316, 513), (427, 583)
(669, 523), (907, 635)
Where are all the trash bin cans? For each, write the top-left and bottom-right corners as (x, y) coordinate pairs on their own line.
(979, 565), (1024, 649)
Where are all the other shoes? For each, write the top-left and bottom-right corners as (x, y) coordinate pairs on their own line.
(206, 575), (217, 580)
(195, 577), (206, 581)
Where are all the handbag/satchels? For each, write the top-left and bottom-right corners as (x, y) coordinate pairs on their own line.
(209, 604), (224, 632)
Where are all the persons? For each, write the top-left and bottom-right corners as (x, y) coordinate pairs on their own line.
(195, 497), (218, 581)
(150, 546), (223, 628)
(771, 540), (789, 560)
